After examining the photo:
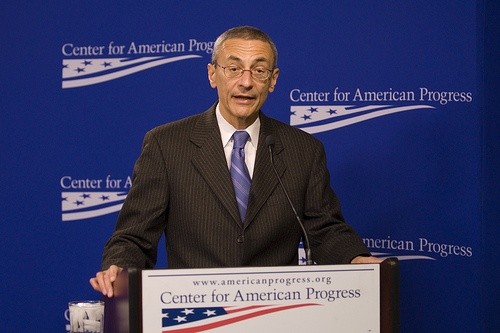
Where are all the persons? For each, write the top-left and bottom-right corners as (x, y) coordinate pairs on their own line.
(88, 25), (388, 298)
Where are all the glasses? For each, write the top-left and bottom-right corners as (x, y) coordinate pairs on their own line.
(215, 63), (274, 80)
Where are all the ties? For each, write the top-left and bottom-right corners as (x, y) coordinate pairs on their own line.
(229, 131), (251, 222)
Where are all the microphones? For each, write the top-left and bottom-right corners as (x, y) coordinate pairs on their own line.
(265, 135), (313, 266)
